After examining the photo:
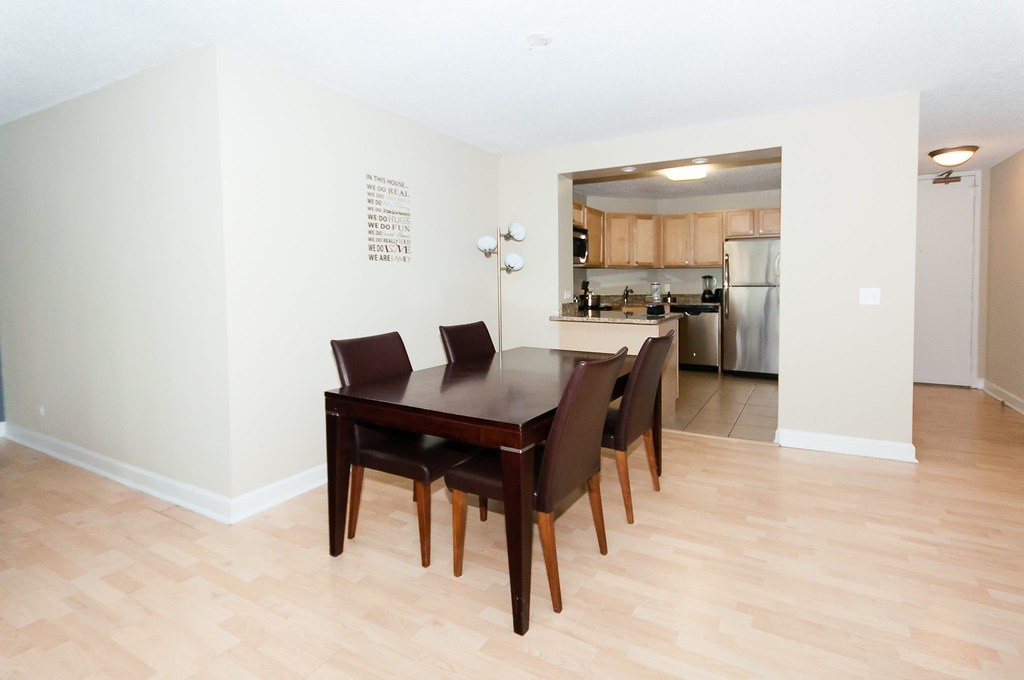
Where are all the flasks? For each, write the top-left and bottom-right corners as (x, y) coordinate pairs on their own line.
(652, 282), (662, 304)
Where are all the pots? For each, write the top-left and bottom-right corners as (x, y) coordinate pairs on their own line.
(577, 280), (601, 308)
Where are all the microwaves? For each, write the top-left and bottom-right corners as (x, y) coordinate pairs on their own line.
(573, 224), (589, 265)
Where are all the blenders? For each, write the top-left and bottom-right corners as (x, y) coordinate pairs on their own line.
(700, 275), (717, 303)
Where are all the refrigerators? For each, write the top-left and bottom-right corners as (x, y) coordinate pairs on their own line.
(721, 236), (781, 380)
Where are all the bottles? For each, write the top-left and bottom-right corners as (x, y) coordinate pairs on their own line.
(667, 291), (672, 305)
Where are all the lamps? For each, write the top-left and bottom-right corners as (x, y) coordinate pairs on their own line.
(928, 145), (979, 166)
(663, 166), (705, 181)
(475, 221), (527, 353)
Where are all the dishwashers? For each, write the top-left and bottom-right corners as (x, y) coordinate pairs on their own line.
(673, 305), (719, 373)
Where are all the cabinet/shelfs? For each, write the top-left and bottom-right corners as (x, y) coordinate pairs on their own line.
(573, 204), (780, 270)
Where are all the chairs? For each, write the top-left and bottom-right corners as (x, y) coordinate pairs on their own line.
(329, 331), (489, 569)
(441, 345), (630, 614)
(437, 321), (497, 364)
(542, 328), (675, 525)
